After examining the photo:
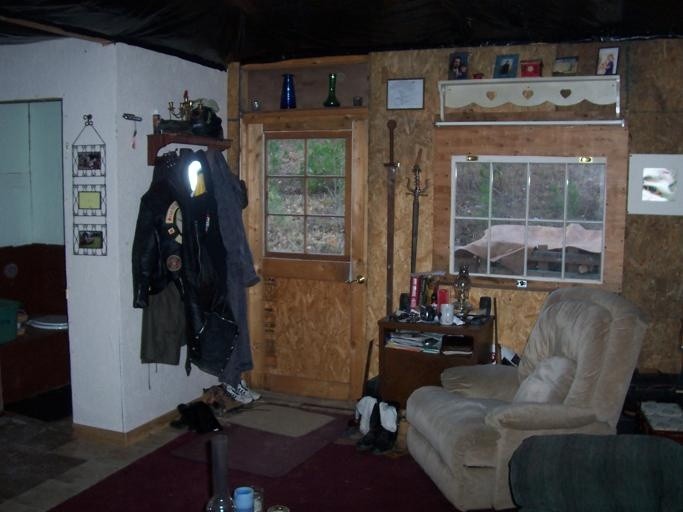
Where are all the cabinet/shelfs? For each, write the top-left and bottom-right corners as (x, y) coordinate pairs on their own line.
(377, 310), (495, 410)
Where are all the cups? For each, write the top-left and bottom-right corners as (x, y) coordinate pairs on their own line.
(440, 303), (453, 325)
(353, 96), (362, 108)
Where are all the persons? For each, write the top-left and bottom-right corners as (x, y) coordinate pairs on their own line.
(604, 53), (616, 75)
(500, 58), (510, 73)
(447, 54), (464, 78)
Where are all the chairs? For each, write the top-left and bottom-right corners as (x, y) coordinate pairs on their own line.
(402, 285), (647, 512)
(0, 241), (70, 402)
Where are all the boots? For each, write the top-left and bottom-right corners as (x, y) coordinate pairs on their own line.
(354, 396), (399, 455)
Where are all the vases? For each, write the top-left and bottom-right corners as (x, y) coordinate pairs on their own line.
(278, 73), (298, 110)
(322, 71), (341, 109)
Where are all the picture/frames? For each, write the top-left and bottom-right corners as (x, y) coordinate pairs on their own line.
(492, 53), (518, 77)
(385, 78), (426, 111)
(594, 45), (621, 76)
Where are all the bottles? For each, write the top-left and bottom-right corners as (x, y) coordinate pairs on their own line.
(206, 434), (264, 512)
(418, 277), (427, 306)
(438, 289), (448, 304)
(280, 73), (297, 109)
(400, 293), (410, 310)
(323, 73), (340, 107)
(430, 278), (442, 310)
(479, 296), (490, 316)
(250, 96), (260, 111)
(266, 505), (289, 512)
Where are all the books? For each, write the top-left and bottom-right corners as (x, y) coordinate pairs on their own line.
(384, 329), (474, 359)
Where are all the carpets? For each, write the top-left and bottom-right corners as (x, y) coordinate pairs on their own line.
(10, 383), (72, 424)
(158, 401), (350, 481)
(223, 402), (337, 442)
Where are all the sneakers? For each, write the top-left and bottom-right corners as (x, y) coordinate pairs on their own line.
(202, 376), (261, 412)
(169, 402), (223, 435)
